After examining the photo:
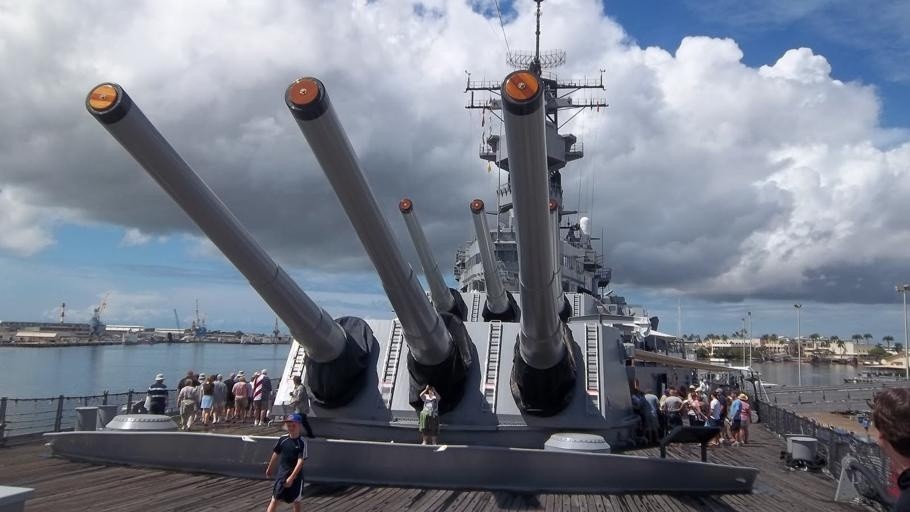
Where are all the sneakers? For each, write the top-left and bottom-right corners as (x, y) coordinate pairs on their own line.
(718, 437), (745, 447)
(180, 416), (266, 430)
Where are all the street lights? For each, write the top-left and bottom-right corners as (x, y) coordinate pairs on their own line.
(793, 303), (803, 386)
(748, 311), (753, 367)
(895, 284), (910, 380)
(741, 317), (746, 365)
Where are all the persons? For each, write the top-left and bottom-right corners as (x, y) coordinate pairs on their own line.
(176, 367), (274, 430)
(146, 372), (169, 414)
(870, 386), (910, 512)
(263, 413), (309, 511)
(288, 375), (316, 438)
(632, 380), (752, 448)
(864, 418), (869, 431)
(418, 384), (442, 445)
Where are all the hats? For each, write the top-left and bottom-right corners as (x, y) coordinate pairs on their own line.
(737, 393), (748, 401)
(155, 373), (165, 380)
(199, 373), (206, 380)
(280, 414), (301, 423)
(237, 371), (244, 376)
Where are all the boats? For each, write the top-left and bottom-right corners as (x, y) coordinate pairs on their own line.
(709, 358), (730, 363)
(843, 365), (910, 386)
(703, 365), (780, 391)
